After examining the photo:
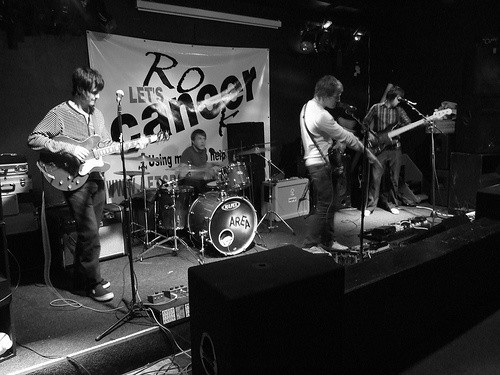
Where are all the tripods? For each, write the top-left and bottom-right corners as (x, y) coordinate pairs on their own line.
(95, 113), (158, 342)
(125, 161), (203, 267)
(404, 103), (448, 229)
(252, 154), (295, 235)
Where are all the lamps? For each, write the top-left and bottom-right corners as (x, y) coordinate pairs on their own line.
(298, 20), (364, 57)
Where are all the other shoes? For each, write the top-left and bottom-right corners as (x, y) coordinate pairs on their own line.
(390, 207), (400, 214)
(320, 241), (351, 253)
(364, 210), (371, 216)
(302, 245), (333, 256)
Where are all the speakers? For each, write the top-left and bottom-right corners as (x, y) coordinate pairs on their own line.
(423, 217), (500, 332)
(475, 184), (500, 219)
(187, 244), (345, 375)
(342, 242), (453, 375)
(261, 178), (309, 221)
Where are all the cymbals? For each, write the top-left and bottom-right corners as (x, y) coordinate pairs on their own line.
(237, 147), (276, 156)
(112, 171), (150, 175)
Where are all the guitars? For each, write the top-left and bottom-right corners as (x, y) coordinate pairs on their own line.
(368, 109), (452, 156)
(327, 146), (347, 173)
(39, 131), (169, 192)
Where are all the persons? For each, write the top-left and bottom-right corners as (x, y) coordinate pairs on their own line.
(300, 75), (376, 256)
(28, 67), (149, 301)
(362, 85), (431, 215)
(180, 129), (220, 194)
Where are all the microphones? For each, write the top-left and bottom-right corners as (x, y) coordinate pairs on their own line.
(398, 97), (411, 104)
(115, 89), (125, 115)
(344, 104), (356, 110)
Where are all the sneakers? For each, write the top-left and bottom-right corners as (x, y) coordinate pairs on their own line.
(86, 279), (115, 301)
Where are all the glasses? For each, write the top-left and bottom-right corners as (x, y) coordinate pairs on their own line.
(83, 87), (99, 94)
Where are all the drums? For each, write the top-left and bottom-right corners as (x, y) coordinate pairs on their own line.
(156, 188), (187, 231)
(188, 191), (257, 256)
(223, 162), (251, 194)
(206, 181), (225, 192)
